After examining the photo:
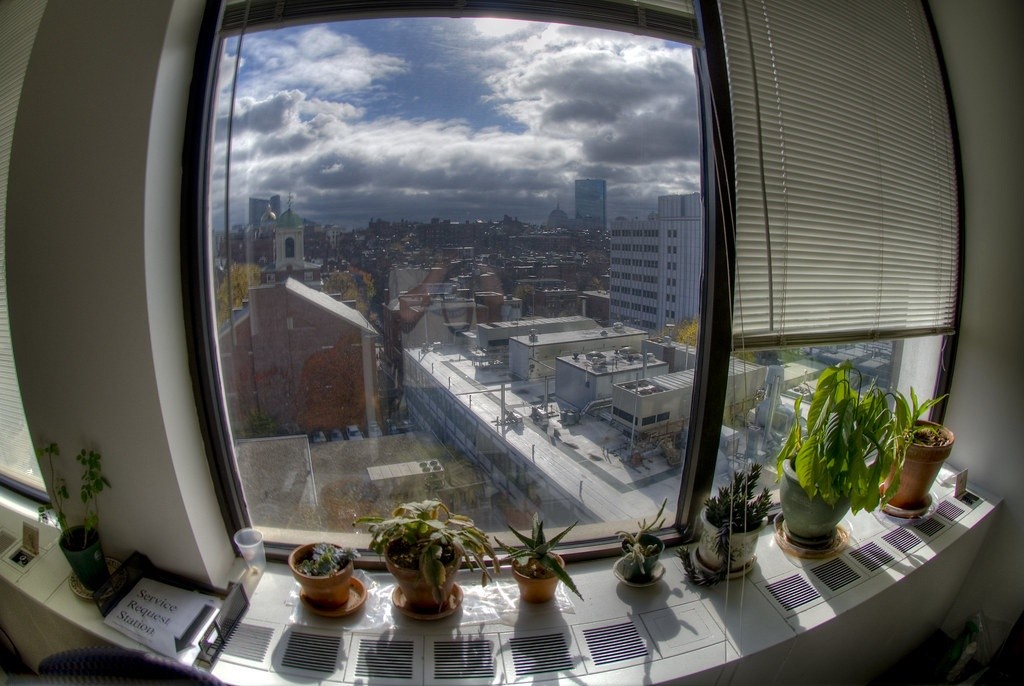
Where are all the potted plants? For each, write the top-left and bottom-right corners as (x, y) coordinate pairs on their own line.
(33, 442), (114, 591)
(613, 499), (670, 587)
(769, 358), (950, 561)
(353, 497), (503, 622)
(877, 387), (958, 517)
(674, 463), (769, 587)
(287, 541), (367, 617)
(488, 510), (586, 603)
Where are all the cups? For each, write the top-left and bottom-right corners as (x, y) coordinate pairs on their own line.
(233, 526), (267, 574)
